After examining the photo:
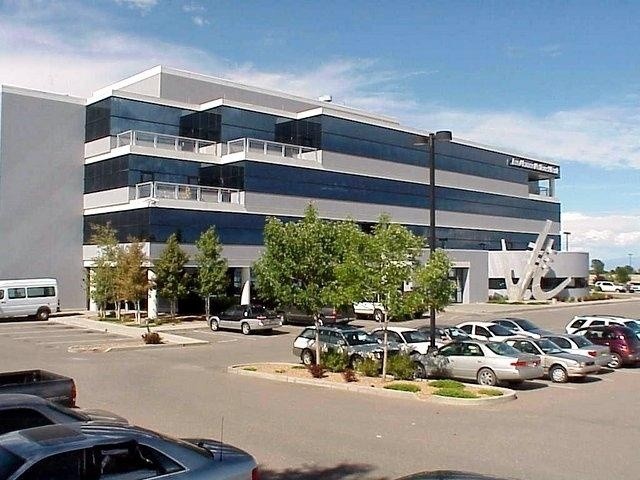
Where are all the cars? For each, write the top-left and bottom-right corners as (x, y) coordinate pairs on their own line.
(211, 305), (282, 335)
(570, 325), (640, 368)
(454, 321), (534, 349)
(409, 339), (544, 388)
(0, 369), (262, 478)
(419, 325), (472, 341)
(370, 326), (446, 356)
(593, 279), (640, 293)
(541, 334), (612, 367)
(502, 337), (598, 383)
(486, 317), (553, 336)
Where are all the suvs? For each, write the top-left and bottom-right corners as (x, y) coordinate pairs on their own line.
(565, 314), (640, 339)
(292, 327), (384, 372)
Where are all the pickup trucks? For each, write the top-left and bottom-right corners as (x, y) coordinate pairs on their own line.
(352, 290), (429, 322)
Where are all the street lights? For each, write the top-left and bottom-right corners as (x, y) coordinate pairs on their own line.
(412, 130), (452, 351)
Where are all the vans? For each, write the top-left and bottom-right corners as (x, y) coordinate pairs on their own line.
(275, 301), (357, 326)
(0, 277), (60, 323)
